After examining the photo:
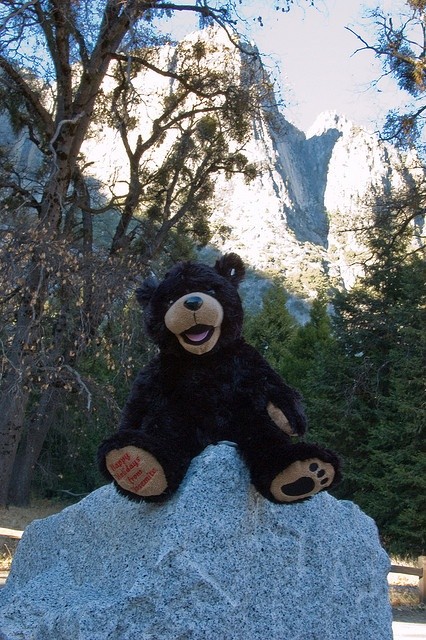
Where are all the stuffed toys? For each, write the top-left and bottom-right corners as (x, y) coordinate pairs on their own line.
(97, 252), (344, 504)
